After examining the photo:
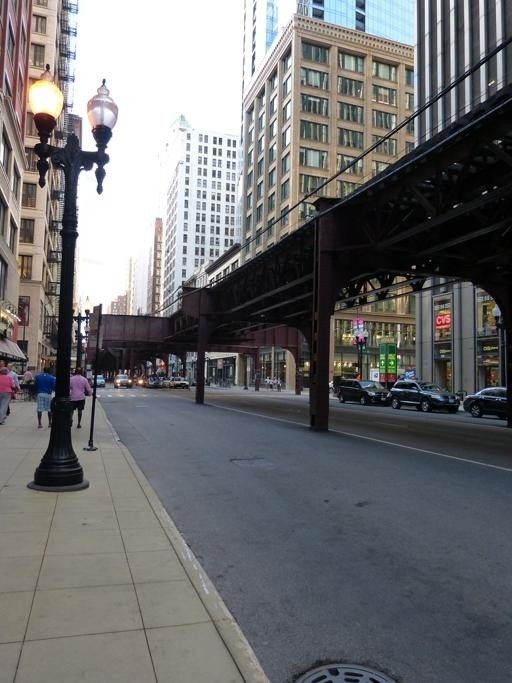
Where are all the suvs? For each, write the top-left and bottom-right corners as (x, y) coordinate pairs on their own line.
(387, 380), (461, 412)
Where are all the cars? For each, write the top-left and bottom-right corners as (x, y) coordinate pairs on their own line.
(463, 387), (508, 419)
(136, 376), (197, 389)
(91, 374), (105, 387)
(114, 374), (132, 388)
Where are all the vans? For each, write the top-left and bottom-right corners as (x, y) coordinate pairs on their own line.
(338, 379), (390, 405)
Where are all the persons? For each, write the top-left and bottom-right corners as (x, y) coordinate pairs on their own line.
(21, 369), (32, 384)
(264, 376), (282, 392)
(0, 359), (21, 425)
(33, 367), (55, 428)
(70, 367), (93, 428)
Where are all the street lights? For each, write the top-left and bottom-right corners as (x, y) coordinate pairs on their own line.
(73, 296), (90, 366)
(355, 328), (368, 380)
(491, 304), (506, 387)
(31, 65), (117, 487)
